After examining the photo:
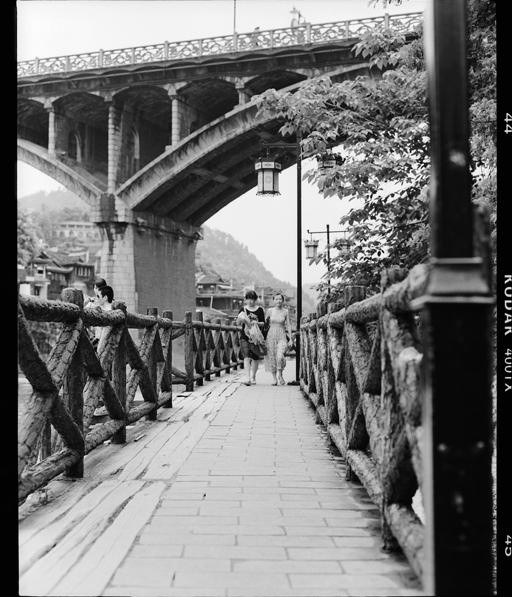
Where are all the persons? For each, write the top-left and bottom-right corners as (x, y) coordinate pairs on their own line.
(263, 293), (293, 386)
(81, 286), (118, 417)
(235, 290), (266, 386)
(82, 278), (107, 348)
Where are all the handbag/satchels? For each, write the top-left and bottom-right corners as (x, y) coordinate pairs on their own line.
(261, 315), (271, 340)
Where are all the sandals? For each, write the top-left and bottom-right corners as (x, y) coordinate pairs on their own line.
(244, 376), (286, 386)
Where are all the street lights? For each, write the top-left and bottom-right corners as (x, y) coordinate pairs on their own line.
(304, 225), (350, 294)
(255, 131), (301, 385)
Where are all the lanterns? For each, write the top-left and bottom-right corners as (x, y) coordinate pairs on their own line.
(317, 153), (346, 194)
(254, 152), (281, 197)
(304, 239), (320, 258)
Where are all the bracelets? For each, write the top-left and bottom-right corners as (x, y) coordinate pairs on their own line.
(88, 297), (92, 302)
